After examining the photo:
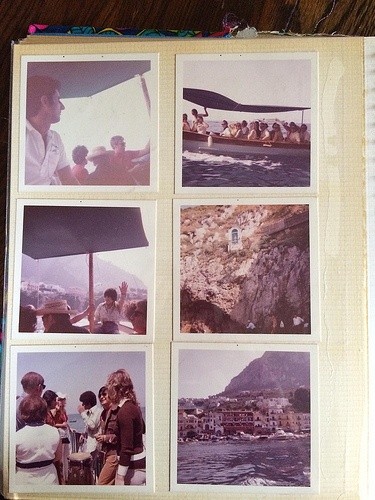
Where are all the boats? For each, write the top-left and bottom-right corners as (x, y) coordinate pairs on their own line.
(183, 128), (310, 150)
(231, 228), (239, 244)
(177, 426), (310, 444)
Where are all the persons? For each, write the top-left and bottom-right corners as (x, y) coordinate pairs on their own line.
(110, 136), (150, 183)
(183, 109), (310, 146)
(245, 318), (257, 332)
(292, 313), (305, 333)
(26, 78), (82, 185)
(15, 369), (146, 485)
(17, 282), (147, 335)
(84, 145), (132, 184)
(65, 143), (92, 183)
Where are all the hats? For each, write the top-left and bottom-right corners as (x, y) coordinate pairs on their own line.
(36, 299), (78, 315)
(57, 391), (66, 399)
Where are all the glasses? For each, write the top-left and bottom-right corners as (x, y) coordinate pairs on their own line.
(40, 384), (46, 389)
(118, 142), (126, 147)
(41, 316), (48, 321)
(98, 393), (109, 398)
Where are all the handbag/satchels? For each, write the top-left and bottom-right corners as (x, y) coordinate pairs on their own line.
(90, 449), (107, 478)
